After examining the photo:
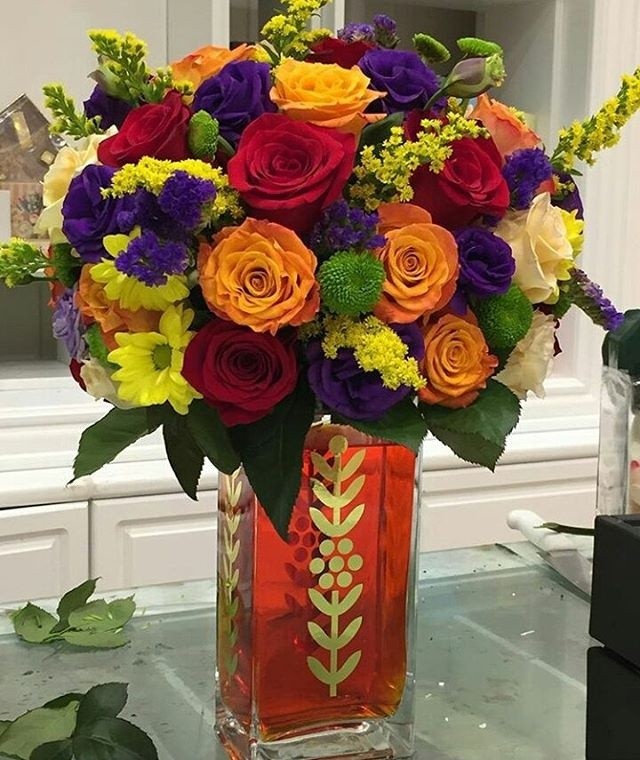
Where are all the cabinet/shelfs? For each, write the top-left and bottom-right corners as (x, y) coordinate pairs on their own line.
(0, 363), (619, 612)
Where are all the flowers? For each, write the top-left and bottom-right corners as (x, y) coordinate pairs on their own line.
(0, 0), (640, 477)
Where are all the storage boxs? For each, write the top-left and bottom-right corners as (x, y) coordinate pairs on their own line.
(580, 510), (639, 760)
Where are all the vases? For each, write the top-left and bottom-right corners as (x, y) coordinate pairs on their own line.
(207, 444), (425, 760)
(594, 352), (640, 566)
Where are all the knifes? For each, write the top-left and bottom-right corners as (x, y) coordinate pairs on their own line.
(506, 510), (594, 596)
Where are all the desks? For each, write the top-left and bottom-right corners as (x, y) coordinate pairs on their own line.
(0, 524), (640, 760)
